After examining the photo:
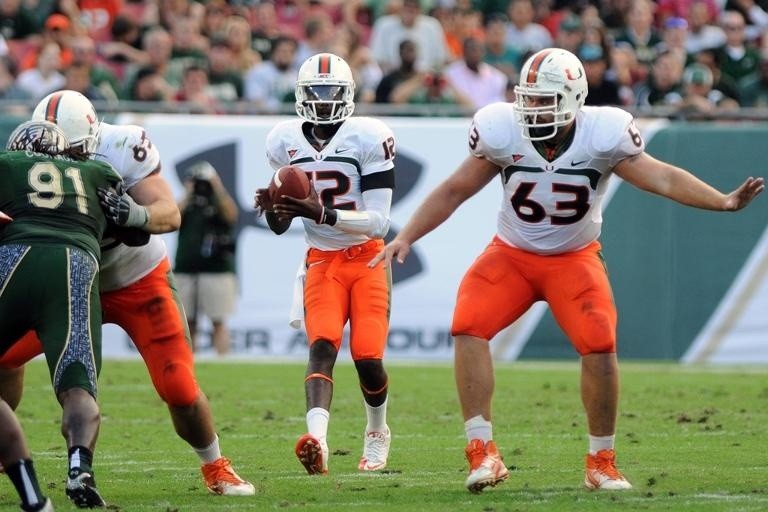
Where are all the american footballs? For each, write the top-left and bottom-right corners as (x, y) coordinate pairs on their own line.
(269, 166), (310, 203)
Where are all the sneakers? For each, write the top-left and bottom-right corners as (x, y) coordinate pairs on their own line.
(358, 425), (391, 472)
(584, 450), (632, 490)
(464, 439), (509, 490)
(295, 433), (328, 474)
(200, 456), (255, 497)
(63, 467), (104, 508)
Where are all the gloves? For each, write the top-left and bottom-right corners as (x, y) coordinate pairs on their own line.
(96, 181), (146, 229)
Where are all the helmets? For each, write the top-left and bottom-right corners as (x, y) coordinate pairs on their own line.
(514, 49), (589, 142)
(31, 90), (99, 154)
(294, 54), (356, 126)
(7, 121), (70, 154)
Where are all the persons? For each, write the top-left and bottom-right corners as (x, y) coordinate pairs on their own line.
(365, 43), (767, 495)
(1, 3), (768, 128)
(253, 54), (397, 478)
(0, 118), (152, 512)
(0, 89), (257, 499)
(167, 162), (240, 356)
